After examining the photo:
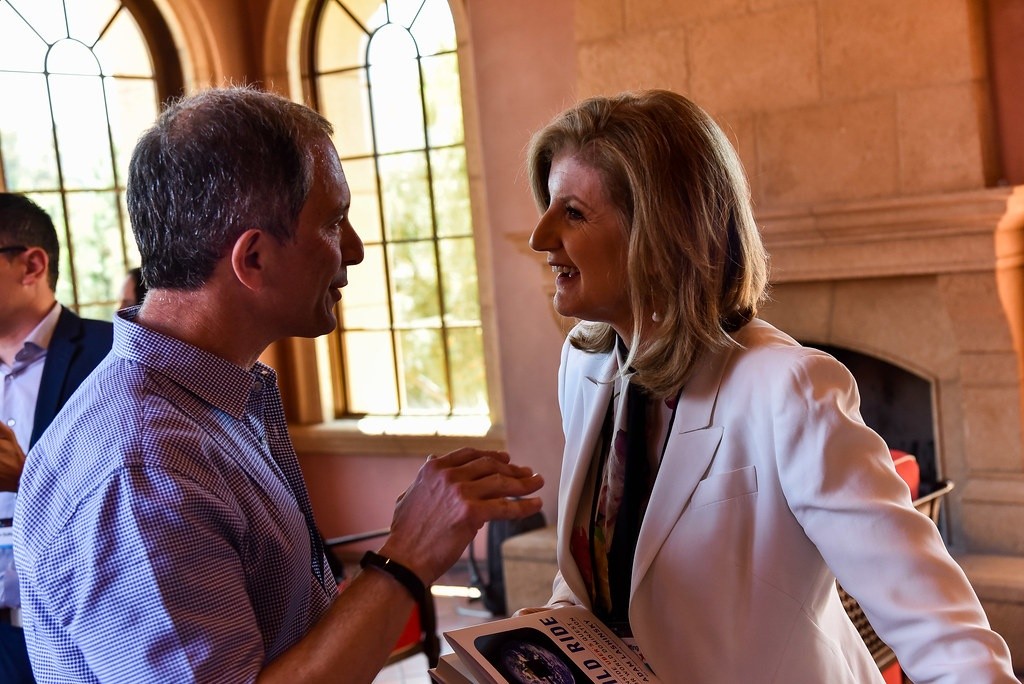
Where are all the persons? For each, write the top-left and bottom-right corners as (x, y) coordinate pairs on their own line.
(11, 88), (545, 684)
(1, 190), (147, 684)
(510, 90), (989, 684)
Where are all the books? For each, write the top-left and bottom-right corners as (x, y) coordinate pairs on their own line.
(428, 602), (662, 684)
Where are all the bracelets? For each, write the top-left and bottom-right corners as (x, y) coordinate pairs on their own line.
(360, 549), (429, 605)
